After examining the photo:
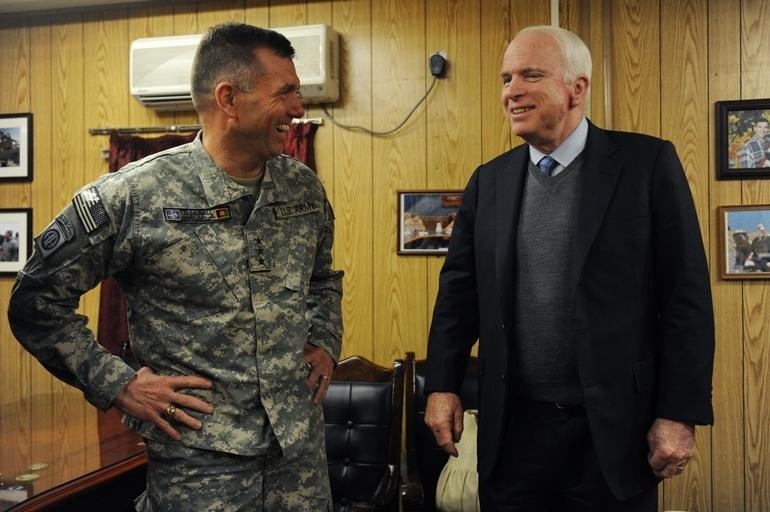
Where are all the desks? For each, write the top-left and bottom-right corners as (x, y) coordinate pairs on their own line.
(0, 393), (148, 512)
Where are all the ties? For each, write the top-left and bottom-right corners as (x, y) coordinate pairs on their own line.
(538, 156), (558, 176)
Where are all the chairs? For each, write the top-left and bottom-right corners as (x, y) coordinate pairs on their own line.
(319, 352), (425, 512)
(413, 355), (479, 512)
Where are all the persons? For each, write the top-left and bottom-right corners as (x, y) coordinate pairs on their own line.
(733, 222), (767, 263)
(423, 23), (719, 510)
(7, 21), (344, 512)
(1, 230), (19, 262)
(728, 117), (770, 169)
(743, 234), (770, 272)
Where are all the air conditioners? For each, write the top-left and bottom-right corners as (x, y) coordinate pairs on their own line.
(129, 24), (340, 113)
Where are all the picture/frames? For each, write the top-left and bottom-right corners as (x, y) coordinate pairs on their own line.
(717, 205), (770, 281)
(0, 113), (33, 183)
(0, 208), (33, 276)
(716, 98), (770, 180)
(396, 190), (466, 255)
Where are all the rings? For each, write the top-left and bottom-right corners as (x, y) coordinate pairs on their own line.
(321, 374), (328, 381)
(167, 405), (176, 416)
(678, 466), (685, 470)
